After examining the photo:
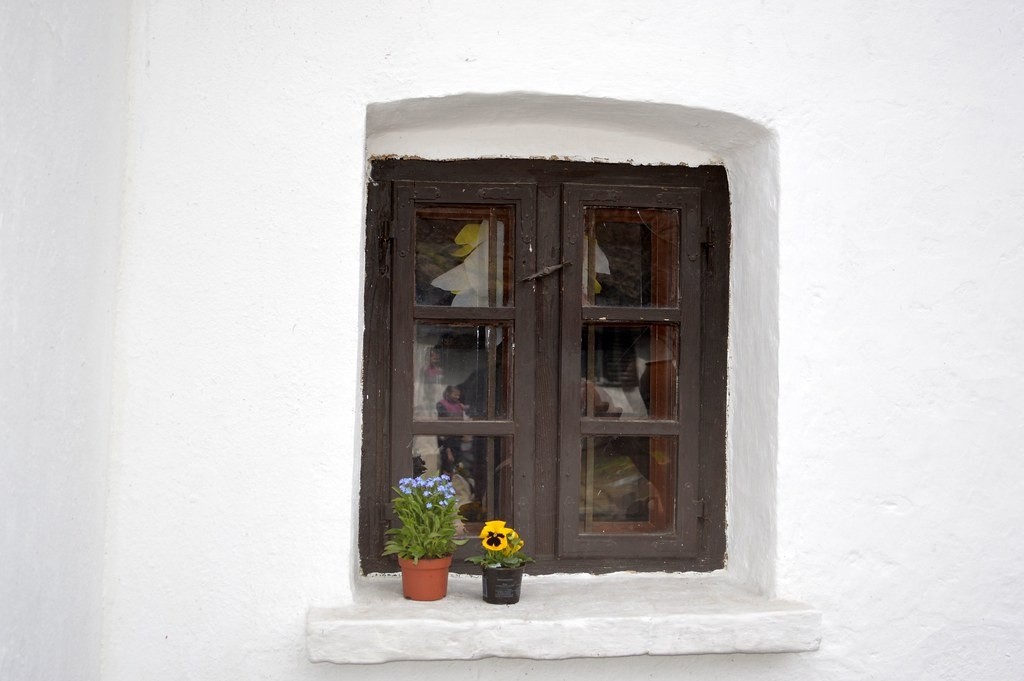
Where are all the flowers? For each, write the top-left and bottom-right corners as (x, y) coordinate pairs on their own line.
(380, 470), (470, 564)
(462, 519), (536, 568)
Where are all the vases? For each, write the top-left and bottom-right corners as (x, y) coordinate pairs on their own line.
(398, 551), (453, 600)
(483, 563), (525, 604)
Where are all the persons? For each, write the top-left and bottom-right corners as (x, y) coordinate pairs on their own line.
(418, 348), (488, 483)
